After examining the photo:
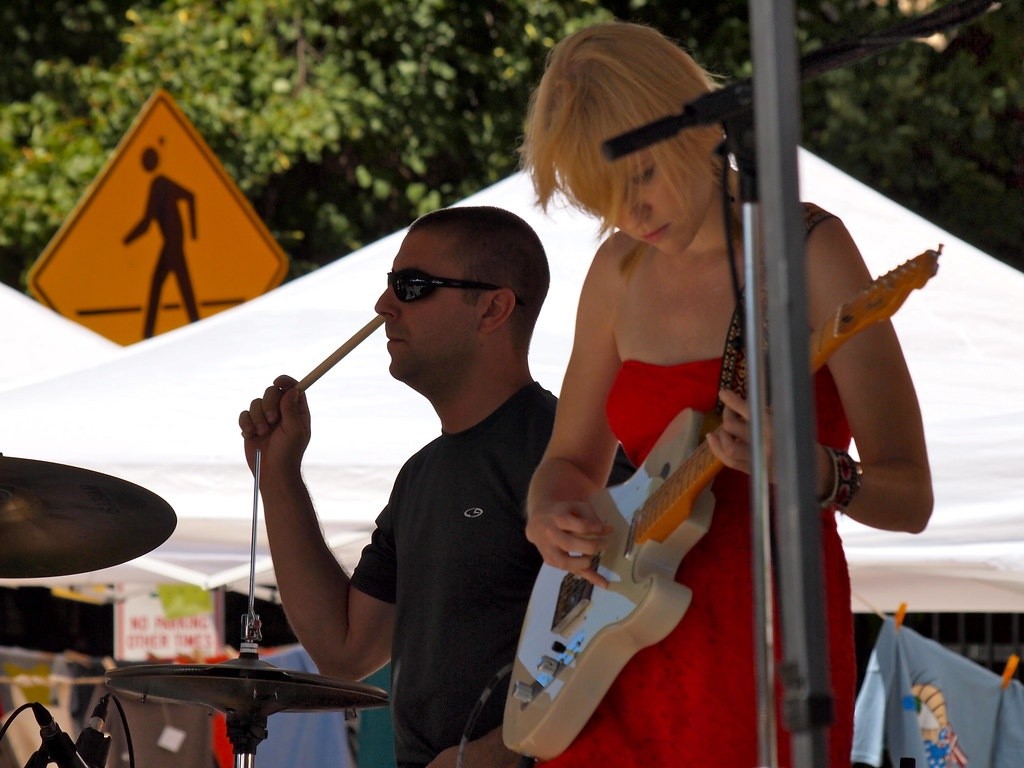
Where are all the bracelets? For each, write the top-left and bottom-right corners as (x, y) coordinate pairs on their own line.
(815, 446), (863, 515)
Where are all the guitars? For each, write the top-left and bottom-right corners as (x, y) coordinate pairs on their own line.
(502, 249), (939, 758)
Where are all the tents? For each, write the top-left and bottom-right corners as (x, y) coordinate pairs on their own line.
(1, 136), (1024, 632)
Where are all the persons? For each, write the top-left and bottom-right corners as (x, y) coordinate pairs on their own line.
(519, 21), (935, 768)
(234, 205), (640, 768)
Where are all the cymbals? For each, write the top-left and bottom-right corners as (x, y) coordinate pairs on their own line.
(0, 453), (180, 580)
(105, 655), (391, 715)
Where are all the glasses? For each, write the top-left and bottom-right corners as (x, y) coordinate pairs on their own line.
(386, 267), (527, 306)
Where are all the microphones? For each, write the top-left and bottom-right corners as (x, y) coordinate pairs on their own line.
(76, 693), (110, 768)
(33, 702), (89, 768)
